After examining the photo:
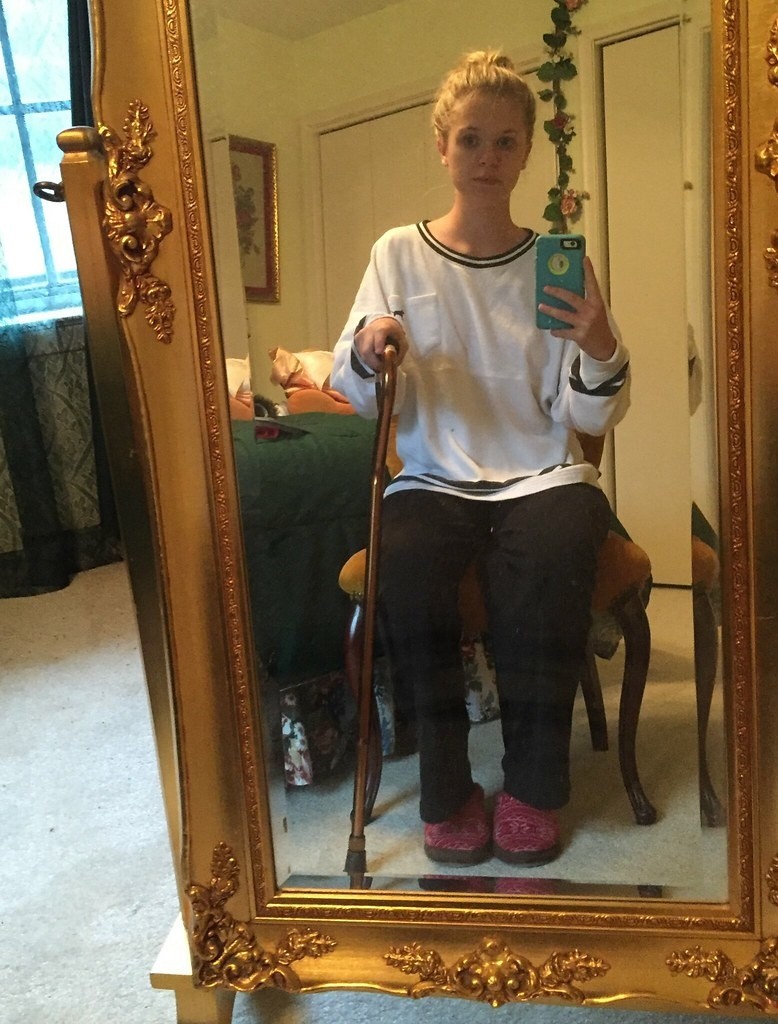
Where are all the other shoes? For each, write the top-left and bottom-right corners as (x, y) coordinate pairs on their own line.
(492, 790), (562, 865)
(424, 782), (491, 864)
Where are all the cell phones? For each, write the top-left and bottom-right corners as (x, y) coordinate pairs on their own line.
(535, 234), (587, 330)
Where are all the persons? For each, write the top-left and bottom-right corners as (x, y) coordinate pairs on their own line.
(329, 48), (631, 866)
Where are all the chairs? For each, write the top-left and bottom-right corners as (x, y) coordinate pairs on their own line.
(339, 418), (657, 832)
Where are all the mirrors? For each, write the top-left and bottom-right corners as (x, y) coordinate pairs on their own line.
(85, 0), (778, 1017)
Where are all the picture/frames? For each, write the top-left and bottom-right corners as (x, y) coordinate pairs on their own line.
(206, 136), (279, 306)
